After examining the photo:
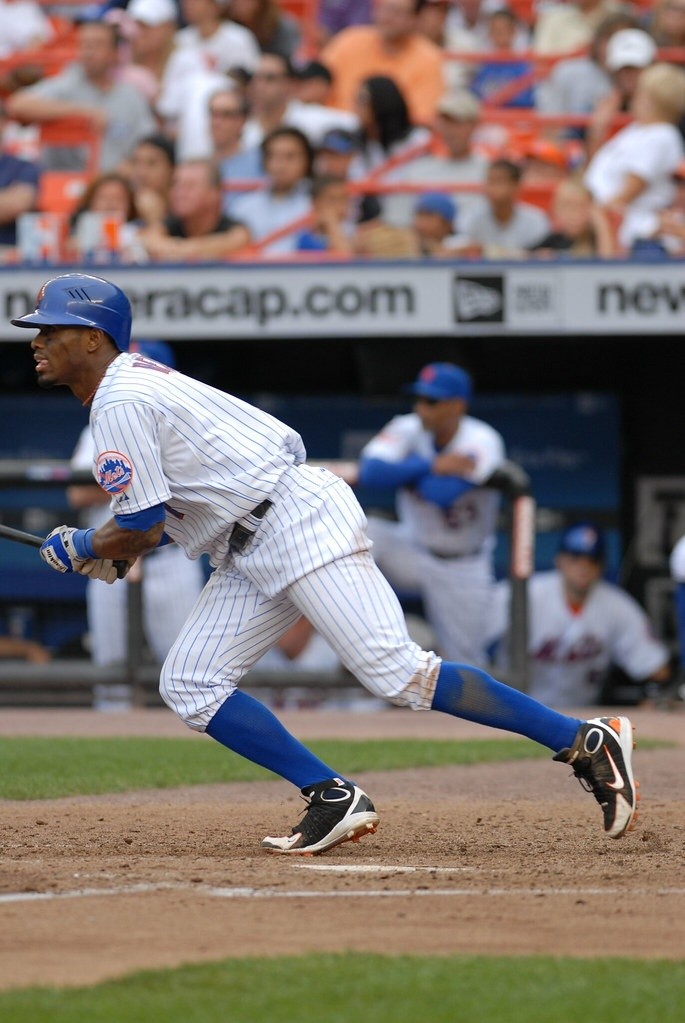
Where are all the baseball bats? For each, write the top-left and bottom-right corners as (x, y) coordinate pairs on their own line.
(0, 523), (128, 579)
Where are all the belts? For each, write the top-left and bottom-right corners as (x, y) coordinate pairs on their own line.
(231, 461), (303, 553)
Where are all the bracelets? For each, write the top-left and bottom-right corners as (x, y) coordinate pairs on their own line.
(82, 528), (100, 560)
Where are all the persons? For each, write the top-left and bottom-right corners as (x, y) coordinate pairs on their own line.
(0, 0), (685, 266)
(493, 522), (685, 713)
(358, 365), (505, 670)
(10, 273), (635, 852)
(67, 341), (202, 715)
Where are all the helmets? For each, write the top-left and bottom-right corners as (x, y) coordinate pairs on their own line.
(11, 272), (132, 354)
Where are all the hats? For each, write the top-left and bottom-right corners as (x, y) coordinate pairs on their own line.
(560, 525), (602, 556)
(409, 362), (472, 401)
(604, 26), (656, 72)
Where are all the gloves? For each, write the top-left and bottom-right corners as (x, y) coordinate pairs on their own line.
(40, 524), (100, 573)
(77, 556), (136, 586)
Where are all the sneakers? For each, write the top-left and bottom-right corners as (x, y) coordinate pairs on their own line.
(262, 778), (380, 855)
(553, 716), (638, 840)
